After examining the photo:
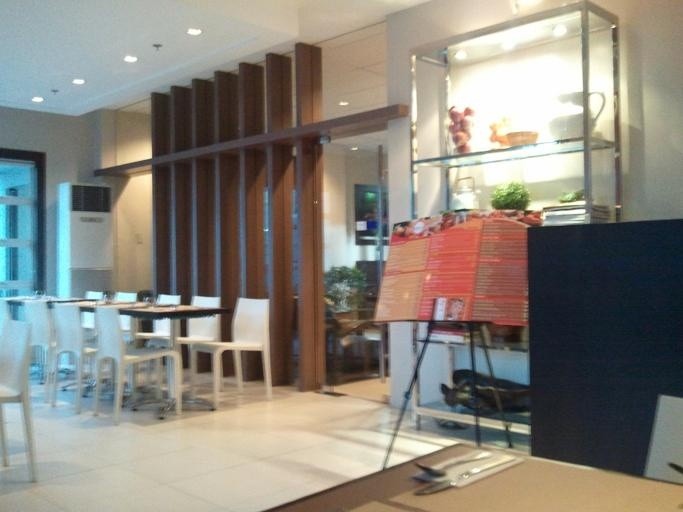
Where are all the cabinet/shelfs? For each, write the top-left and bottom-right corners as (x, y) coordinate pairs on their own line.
(409, 3), (624, 439)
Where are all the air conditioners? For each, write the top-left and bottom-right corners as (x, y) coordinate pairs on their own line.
(56, 183), (114, 296)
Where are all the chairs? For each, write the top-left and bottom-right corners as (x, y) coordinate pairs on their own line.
(26, 289), (275, 424)
(0, 298), (39, 481)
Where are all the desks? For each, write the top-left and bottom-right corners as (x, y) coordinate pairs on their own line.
(257, 443), (683, 512)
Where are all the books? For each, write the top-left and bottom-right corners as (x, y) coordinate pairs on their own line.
(429, 329), (481, 344)
(542, 201), (611, 226)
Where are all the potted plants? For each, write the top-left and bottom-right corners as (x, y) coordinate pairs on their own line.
(324, 265), (366, 319)
